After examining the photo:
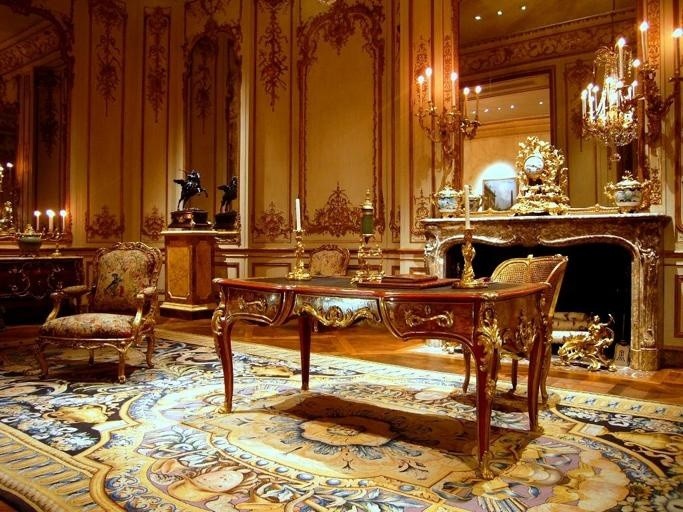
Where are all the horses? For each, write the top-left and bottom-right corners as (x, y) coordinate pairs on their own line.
(172, 168), (209, 211)
(217, 176), (237, 213)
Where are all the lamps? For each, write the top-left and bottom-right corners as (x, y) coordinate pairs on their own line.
(461, 86), (482, 141)
(578, 39), (638, 161)
(617, 21), (682, 144)
(414, 66), (460, 158)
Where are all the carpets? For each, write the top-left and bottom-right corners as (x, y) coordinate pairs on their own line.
(0, 326), (683, 512)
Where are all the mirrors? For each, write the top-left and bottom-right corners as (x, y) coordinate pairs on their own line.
(451, 2), (643, 216)
(461, 63), (557, 213)
(0, 2), (74, 243)
(181, 28), (240, 231)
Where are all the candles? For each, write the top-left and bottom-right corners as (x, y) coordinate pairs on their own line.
(464, 184), (471, 229)
(62, 211), (66, 231)
(296, 198), (302, 231)
(49, 211), (51, 230)
(37, 213), (39, 232)
(51, 210), (53, 231)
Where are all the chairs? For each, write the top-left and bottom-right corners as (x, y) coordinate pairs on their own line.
(463, 256), (568, 403)
(308, 242), (350, 334)
(32, 242), (163, 383)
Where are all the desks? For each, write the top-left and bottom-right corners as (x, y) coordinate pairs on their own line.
(0, 256), (84, 332)
(212, 278), (552, 479)
